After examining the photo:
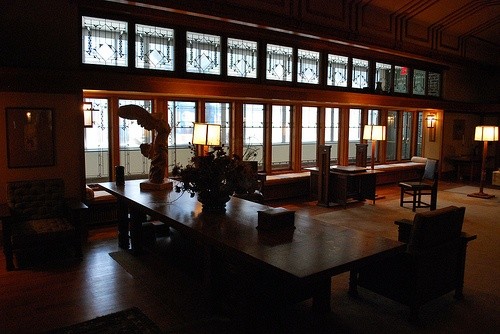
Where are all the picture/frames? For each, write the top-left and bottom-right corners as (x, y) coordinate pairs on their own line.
(5, 106), (57, 168)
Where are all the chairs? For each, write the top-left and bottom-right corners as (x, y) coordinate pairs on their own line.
(0, 177), (89, 271)
(399, 158), (438, 211)
(347, 205), (477, 324)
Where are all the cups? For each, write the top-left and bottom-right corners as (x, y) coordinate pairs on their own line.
(116, 166), (124, 186)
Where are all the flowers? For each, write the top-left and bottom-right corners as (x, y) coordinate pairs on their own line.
(176, 141), (264, 198)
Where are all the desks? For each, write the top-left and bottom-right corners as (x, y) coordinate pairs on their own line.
(99, 178), (406, 329)
(303, 167), (384, 210)
(445, 156), (481, 184)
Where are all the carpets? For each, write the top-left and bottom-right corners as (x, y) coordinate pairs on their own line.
(40, 305), (166, 334)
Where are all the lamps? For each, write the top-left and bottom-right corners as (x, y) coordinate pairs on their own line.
(466, 125), (498, 199)
(363, 125), (387, 206)
(191, 122), (221, 146)
(426, 112), (436, 128)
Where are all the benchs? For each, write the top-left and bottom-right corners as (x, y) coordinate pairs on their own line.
(85, 156), (429, 225)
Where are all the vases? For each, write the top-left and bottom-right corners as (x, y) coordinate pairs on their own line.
(195, 192), (231, 211)
